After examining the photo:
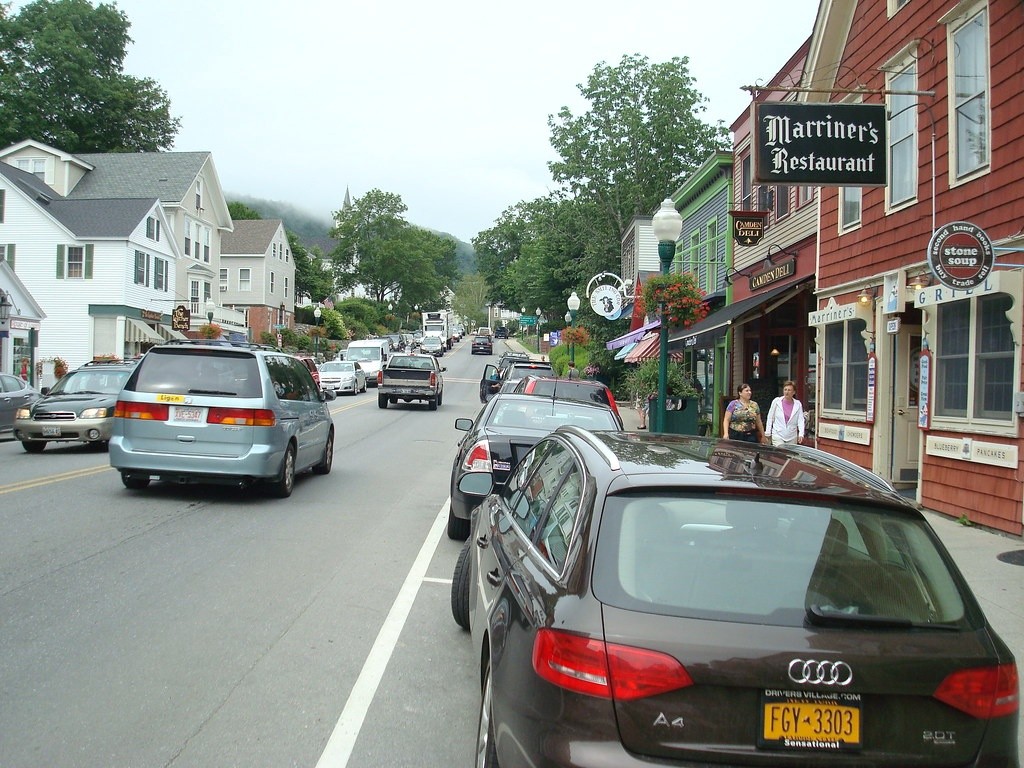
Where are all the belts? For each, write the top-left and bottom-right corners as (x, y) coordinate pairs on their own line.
(729, 428), (755, 435)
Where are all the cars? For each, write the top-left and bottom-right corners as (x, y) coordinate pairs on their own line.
(332, 351), (348, 362)
(300, 356), (323, 390)
(379, 329), (423, 353)
(108, 338), (335, 498)
(0, 371), (46, 434)
(447, 393), (623, 541)
(318, 360), (367, 396)
(13, 357), (140, 453)
(447, 323), (466, 350)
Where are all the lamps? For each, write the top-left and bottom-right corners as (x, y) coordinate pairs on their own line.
(763, 244), (797, 270)
(0, 295), (13, 325)
(723, 267), (749, 287)
(858, 284), (876, 306)
(909, 270), (930, 290)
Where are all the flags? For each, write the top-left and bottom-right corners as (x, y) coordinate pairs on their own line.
(324, 296), (334, 310)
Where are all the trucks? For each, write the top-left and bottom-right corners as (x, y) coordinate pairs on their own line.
(422, 312), (449, 352)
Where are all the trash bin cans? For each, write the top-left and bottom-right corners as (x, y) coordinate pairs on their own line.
(648, 394), (698, 435)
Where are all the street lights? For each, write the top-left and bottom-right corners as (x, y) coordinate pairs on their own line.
(521, 306), (525, 341)
(536, 307), (541, 353)
(206, 296), (217, 324)
(565, 311), (572, 356)
(314, 306), (321, 358)
(566, 292), (581, 367)
(650, 196), (684, 435)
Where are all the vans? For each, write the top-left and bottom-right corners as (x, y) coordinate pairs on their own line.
(477, 327), (494, 337)
(340, 339), (391, 387)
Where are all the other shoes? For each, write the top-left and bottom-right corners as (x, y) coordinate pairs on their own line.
(637, 425), (646, 429)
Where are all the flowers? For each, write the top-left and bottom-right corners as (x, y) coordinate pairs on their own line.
(560, 327), (589, 347)
(635, 272), (709, 327)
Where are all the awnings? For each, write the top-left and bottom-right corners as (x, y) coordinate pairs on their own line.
(125, 318), (165, 344)
(668, 273), (815, 353)
(157, 323), (193, 346)
(614, 342), (638, 360)
(211, 335), (232, 347)
(605, 316), (674, 350)
(624, 332), (684, 363)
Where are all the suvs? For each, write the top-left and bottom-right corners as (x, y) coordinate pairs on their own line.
(495, 327), (509, 339)
(513, 376), (620, 416)
(449, 424), (1019, 768)
(479, 361), (554, 405)
(472, 335), (494, 355)
(420, 335), (446, 357)
(498, 350), (530, 370)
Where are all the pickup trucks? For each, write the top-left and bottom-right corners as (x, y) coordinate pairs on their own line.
(377, 352), (446, 411)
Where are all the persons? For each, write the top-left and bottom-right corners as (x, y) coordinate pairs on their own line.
(638, 390), (649, 430)
(16, 358), (30, 385)
(566, 361), (579, 380)
(766, 380), (804, 445)
(723, 383), (765, 444)
(745, 452), (763, 481)
